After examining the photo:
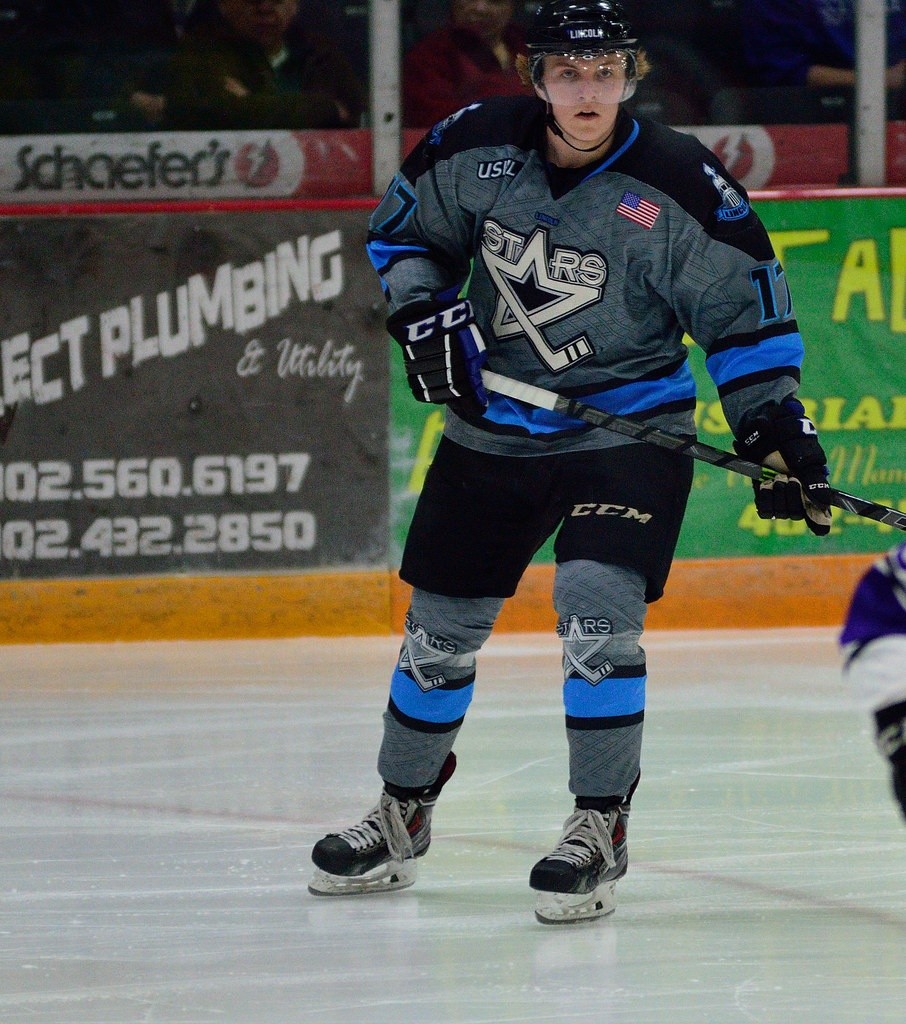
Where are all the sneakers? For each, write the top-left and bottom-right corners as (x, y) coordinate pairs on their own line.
(309, 749), (456, 896)
(530, 768), (640, 925)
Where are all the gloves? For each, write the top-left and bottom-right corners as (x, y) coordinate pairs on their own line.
(386, 298), (489, 420)
(732, 395), (832, 536)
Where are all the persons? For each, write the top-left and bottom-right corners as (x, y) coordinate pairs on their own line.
(308, 0), (833, 925)
(1, 0), (905, 130)
(839, 539), (906, 823)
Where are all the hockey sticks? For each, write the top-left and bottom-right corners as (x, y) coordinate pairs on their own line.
(480, 364), (906, 535)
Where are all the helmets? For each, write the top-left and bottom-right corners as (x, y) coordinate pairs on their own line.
(525, 0), (638, 152)
